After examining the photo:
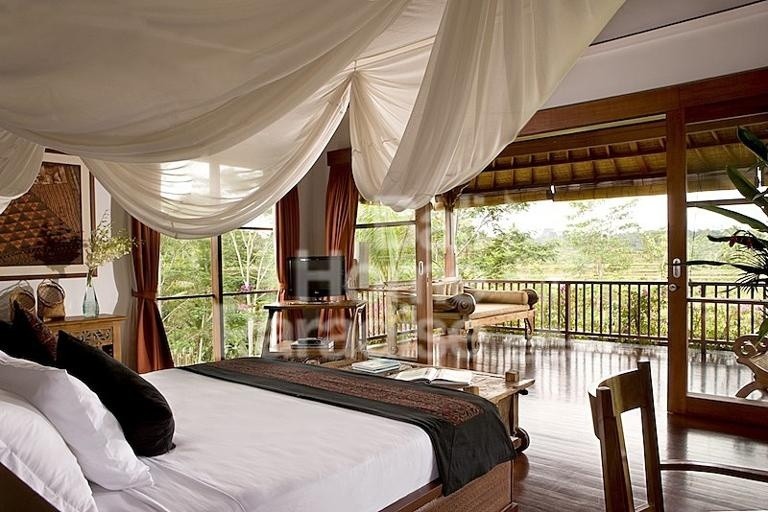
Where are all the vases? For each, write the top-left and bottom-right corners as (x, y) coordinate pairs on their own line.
(83, 281), (98, 319)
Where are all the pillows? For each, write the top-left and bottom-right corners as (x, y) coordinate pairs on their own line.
(432, 279), (464, 295)
(0, 302), (176, 512)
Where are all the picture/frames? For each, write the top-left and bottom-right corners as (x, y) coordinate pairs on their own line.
(0, 148), (98, 281)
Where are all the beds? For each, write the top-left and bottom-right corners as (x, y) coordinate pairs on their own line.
(0, 357), (521, 512)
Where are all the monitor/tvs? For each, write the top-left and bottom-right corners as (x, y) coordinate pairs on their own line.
(286, 256), (345, 304)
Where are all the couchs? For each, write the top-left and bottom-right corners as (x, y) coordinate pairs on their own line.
(392, 288), (539, 355)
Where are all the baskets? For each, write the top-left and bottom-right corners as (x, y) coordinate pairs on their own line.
(37, 280), (66, 319)
(9, 285), (36, 322)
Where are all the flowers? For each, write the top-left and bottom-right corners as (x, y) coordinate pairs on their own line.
(83, 209), (144, 286)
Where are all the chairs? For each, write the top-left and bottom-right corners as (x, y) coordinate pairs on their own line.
(589, 359), (768, 512)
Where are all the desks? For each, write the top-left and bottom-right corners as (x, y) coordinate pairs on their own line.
(261, 300), (366, 359)
